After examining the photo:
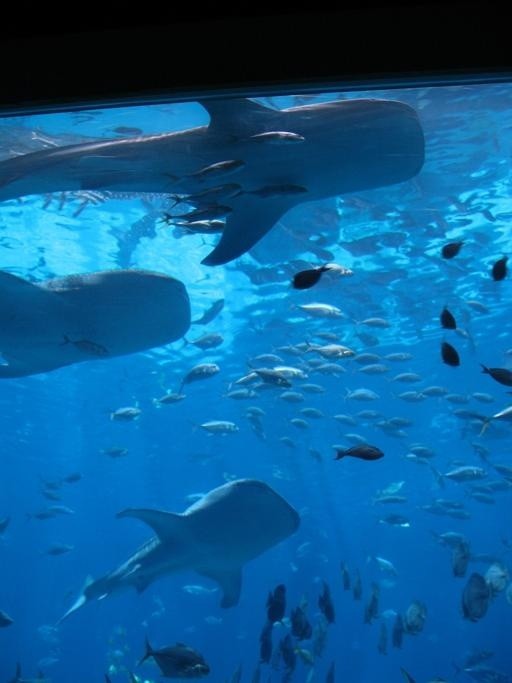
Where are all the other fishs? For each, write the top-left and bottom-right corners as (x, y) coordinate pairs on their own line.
(1, 81), (512, 683)
